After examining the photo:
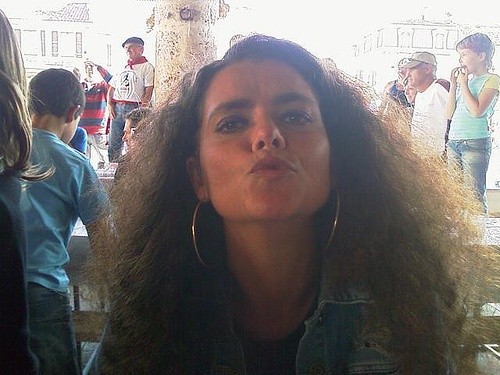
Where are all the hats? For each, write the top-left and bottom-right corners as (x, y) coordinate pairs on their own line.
(401, 50), (437, 69)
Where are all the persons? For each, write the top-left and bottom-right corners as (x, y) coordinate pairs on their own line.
(80, 33), (500, 375)
(382, 33), (500, 217)
(0, 7), (157, 375)
